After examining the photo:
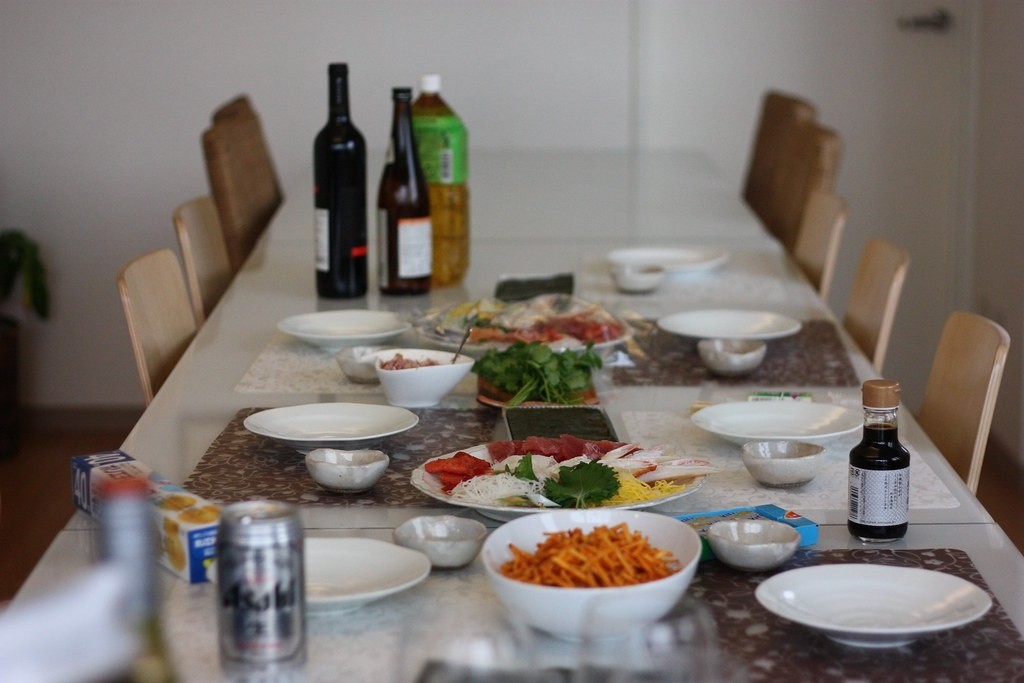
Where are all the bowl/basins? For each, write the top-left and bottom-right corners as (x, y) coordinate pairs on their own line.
(304, 446), (390, 494)
(604, 247), (666, 292)
(375, 348), (475, 408)
(393, 514), (488, 569)
(740, 440), (826, 488)
(707, 519), (802, 572)
(481, 508), (702, 643)
(335, 344), (401, 385)
(698, 339), (768, 379)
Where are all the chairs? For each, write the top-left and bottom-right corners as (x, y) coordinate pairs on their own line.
(742, 88), (1010, 497)
(115, 93), (285, 403)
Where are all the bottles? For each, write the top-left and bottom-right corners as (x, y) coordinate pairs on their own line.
(846, 379), (912, 545)
(312, 61), (369, 300)
(80, 475), (181, 682)
(410, 71), (473, 290)
(375, 85), (434, 298)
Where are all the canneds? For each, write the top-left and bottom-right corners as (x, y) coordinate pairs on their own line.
(215, 500), (306, 671)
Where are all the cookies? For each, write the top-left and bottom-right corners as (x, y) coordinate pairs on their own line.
(156, 494), (220, 571)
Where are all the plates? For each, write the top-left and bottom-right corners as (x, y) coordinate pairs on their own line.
(418, 335), (634, 358)
(754, 564), (993, 648)
(415, 299), (636, 359)
(206, 537), (431, 618)
(408, 444), (708, 523)
(656, 307), (803, 341)
(661, 243), (725, 274)
(243, 402), (419, 456)
(478, 374), (600, 409)
(689, 399), (864, 445)
(276, 309), (412, 351)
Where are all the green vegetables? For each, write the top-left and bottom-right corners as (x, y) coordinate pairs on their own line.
(496, 453), (622, 509)
(461, 312), (512, 333)
(470, 339), (603, 407)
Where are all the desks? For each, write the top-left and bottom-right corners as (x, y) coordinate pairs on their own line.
(1, 148), (1024, 683)
